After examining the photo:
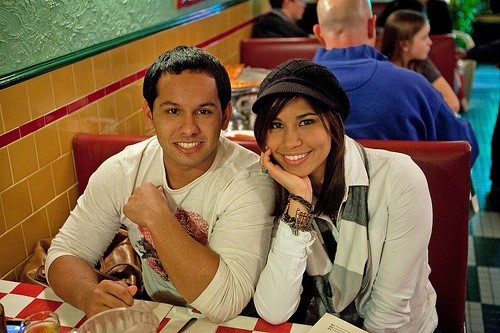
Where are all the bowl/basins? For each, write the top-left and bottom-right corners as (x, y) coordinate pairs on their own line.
(76, 306), (158, 333)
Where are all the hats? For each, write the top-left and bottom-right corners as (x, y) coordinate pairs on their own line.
(252, 58), (350, 121)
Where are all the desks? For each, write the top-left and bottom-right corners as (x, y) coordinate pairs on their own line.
(0, 281), (313, 333)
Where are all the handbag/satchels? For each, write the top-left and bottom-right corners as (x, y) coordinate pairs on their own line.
(19, 228), (142, 288)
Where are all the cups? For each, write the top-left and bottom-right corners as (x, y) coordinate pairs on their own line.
(20, 310), (60, 333)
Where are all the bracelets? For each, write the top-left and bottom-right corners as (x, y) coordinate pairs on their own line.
(282, 194), (314, 232)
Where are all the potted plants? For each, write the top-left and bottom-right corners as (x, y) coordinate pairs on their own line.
(452, 0), (485, 101)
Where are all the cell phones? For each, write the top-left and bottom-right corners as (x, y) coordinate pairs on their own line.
(6, 321), (27, 333)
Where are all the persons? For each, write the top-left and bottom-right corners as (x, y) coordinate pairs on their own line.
(43, 45), (279, 324)
(376, 0), (452, 35)
(378, 9), (459, 113)
(310, 0), (479, 167)
(253, 57), (439, 333)
(251, 0), (309, 39)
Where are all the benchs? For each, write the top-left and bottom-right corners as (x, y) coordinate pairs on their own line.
(73, 132), (472, 333)
(240, 37), (455, 89)
